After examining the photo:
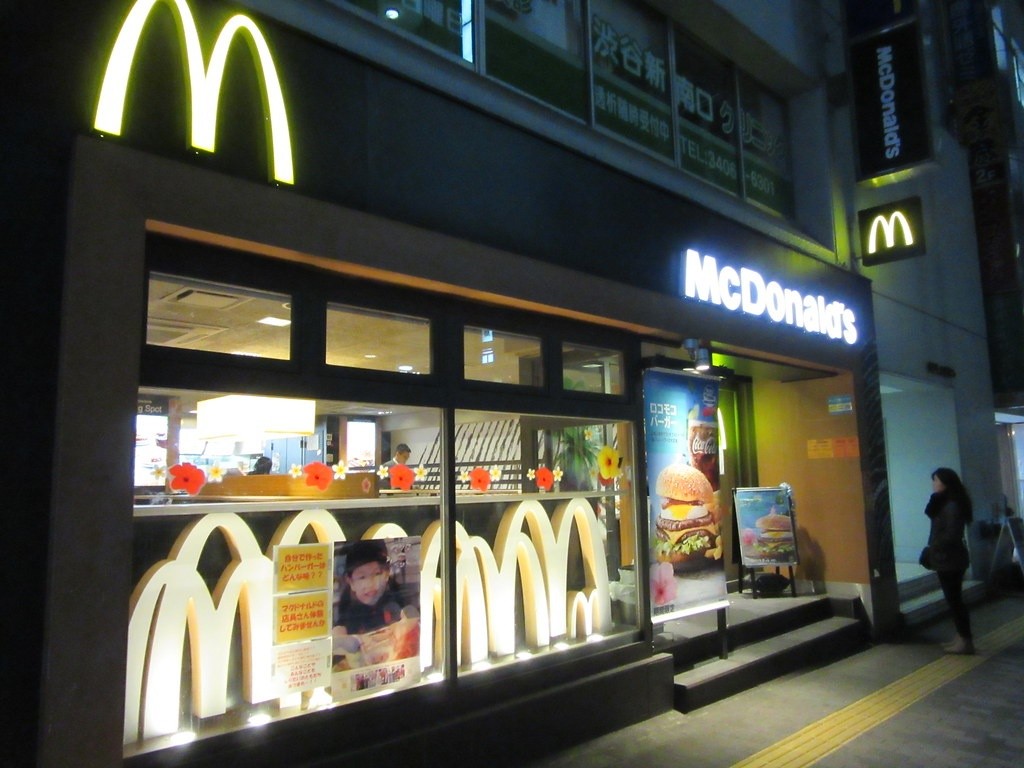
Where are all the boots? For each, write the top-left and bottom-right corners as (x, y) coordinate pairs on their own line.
(941, 634), (974, 654)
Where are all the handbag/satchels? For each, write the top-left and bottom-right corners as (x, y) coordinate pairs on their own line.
(919, 546), (936, 571)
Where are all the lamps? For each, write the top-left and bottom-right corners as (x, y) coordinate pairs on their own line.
(195, 394), (315, 442)
(685, 338), (710, 371)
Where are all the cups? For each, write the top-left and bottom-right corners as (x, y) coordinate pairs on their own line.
(689, 421), (718, 491)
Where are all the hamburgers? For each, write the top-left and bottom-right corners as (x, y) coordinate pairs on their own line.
(754, 514), (796, 557)
(654, 463), (723, 572)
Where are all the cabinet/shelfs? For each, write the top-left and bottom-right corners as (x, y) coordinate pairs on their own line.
(263, 415), (339, 474)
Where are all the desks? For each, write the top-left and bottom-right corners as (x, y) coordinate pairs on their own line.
(378, 488), (519, 495)
(135, 494), (321, 502)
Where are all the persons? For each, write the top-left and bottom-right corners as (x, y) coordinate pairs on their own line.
(247, 457), (272, 476)
(379, 444), (411, 488)
(918, 468), (975, 654)
(331, 540), (412, 666)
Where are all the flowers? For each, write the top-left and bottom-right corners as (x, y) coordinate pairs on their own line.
(650, 561), (678, 605)
(458, 464), (503, 492)
(149, 460), (223, 496)
(289, 459), (350, 492)
(361, 477), (371, 493)
(376, 464), (425, 491)
(526, 466), (564, 491)
(563, 426), (620, 479)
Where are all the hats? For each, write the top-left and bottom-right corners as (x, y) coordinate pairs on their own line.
(346, 540), (387, 575)
